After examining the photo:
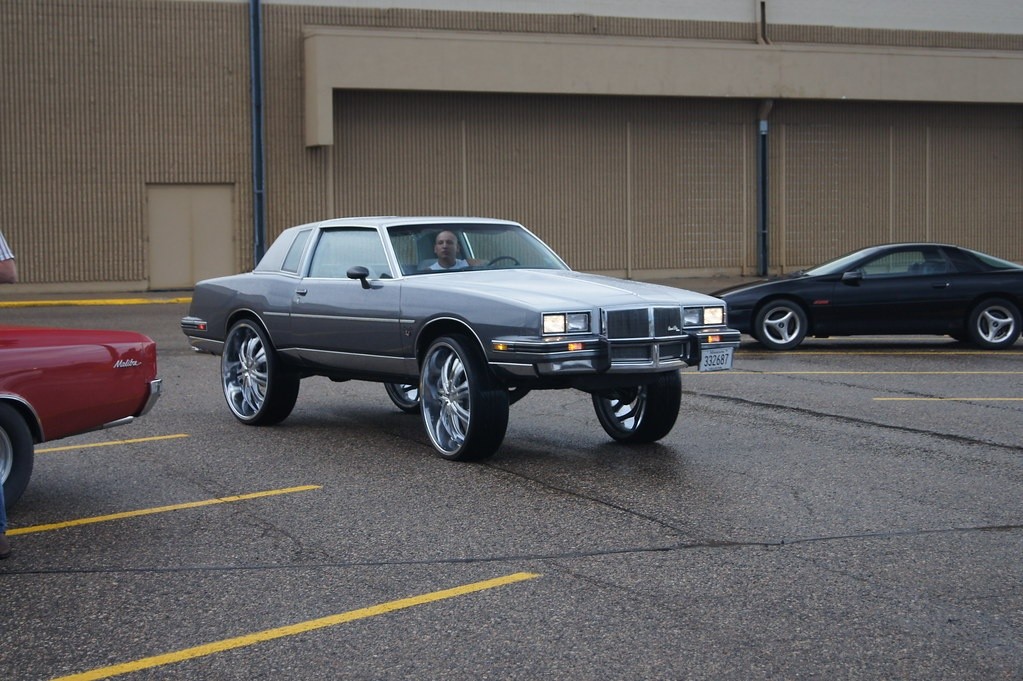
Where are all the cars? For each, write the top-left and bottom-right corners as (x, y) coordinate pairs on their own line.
(706, 243), (1023, 349)
(0, 327), (162, 510)
(181, 216), (740, 463)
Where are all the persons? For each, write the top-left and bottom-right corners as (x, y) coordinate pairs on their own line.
(0, 232), (17, 284)
(423, 231), (488, 271)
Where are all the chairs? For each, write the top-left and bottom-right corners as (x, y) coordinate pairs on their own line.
(415, 234), (437, 269)
(908, 260), (951, 272)
